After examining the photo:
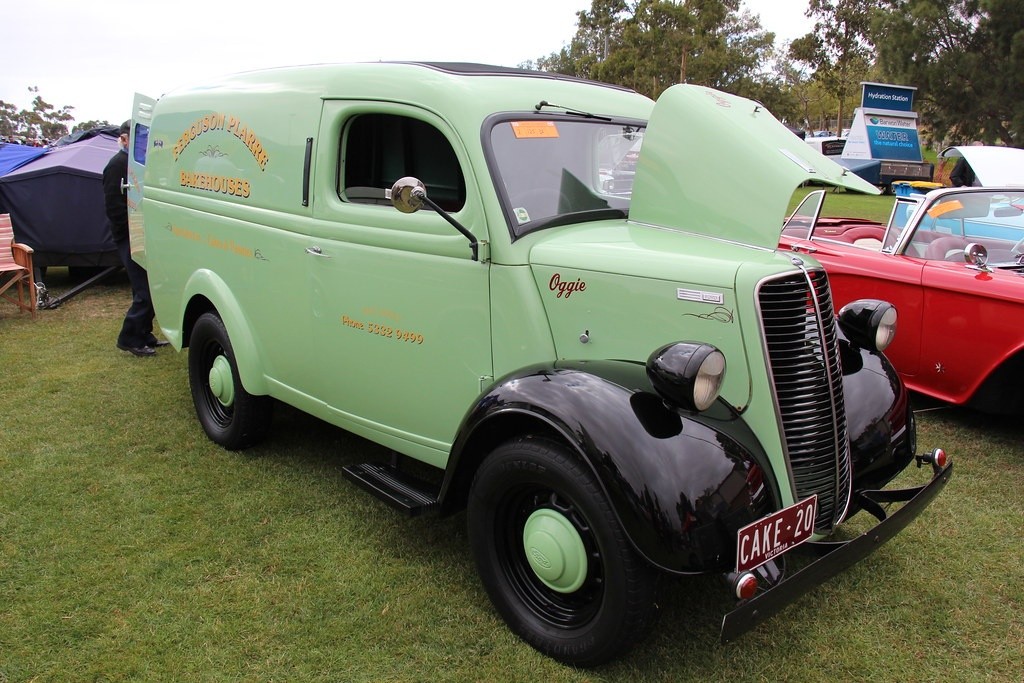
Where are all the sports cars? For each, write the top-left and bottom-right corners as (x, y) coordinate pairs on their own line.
(777, 187), (1024, 416)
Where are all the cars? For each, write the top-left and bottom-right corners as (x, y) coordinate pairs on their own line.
(784, 124), (851, 140)
(120, 60), (954, 668)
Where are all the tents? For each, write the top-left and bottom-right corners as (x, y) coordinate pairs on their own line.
(0, 125), (154, 284)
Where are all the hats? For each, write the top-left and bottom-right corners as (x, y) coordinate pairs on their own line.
(120, 118), (140, 134)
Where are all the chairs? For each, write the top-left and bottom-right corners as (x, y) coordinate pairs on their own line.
(0, 212), (36, 321)
(925, 236), (969, 263)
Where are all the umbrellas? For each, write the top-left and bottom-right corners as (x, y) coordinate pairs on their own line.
(0, 142), (54, 177)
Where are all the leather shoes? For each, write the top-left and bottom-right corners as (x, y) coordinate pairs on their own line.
(116, 340), (156, 357)
(151, 340), (169, 346)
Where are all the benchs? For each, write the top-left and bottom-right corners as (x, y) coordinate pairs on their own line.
(780, 224), (921, 259)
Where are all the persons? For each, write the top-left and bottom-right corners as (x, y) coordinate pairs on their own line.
(0, 134), (55, 149)
(100, 120), (169, 357)
(949, 141), (984, 189)
